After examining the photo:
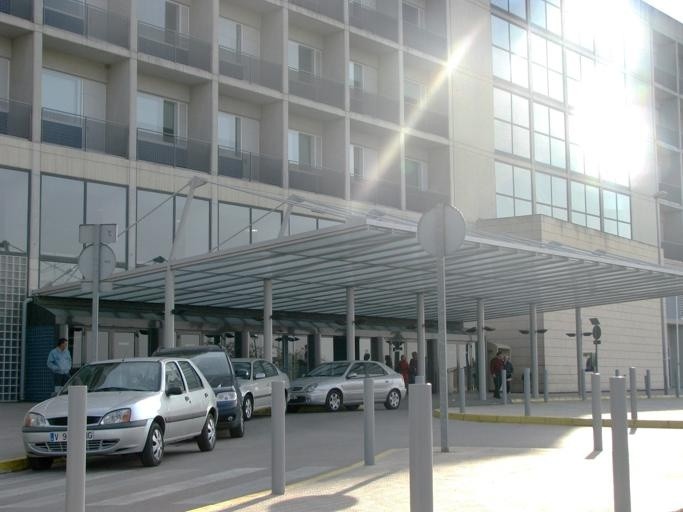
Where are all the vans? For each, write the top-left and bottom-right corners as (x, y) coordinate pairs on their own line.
(152, 343), (245, 438)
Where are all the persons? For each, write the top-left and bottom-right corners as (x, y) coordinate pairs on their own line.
(46, 338), (72, 386)
(398, 355), (409, 386)
(364, 353), (370, 360)
(385, 355), (392, 368)
(410, 352), (417, 384)
(490, 353), (505, 399)
(504, 356), (513, 394)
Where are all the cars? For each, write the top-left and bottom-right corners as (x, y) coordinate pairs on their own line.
(291, 361), (406, 412)
(230, 357), (291, 420)
(22, 357), (219, 470)
